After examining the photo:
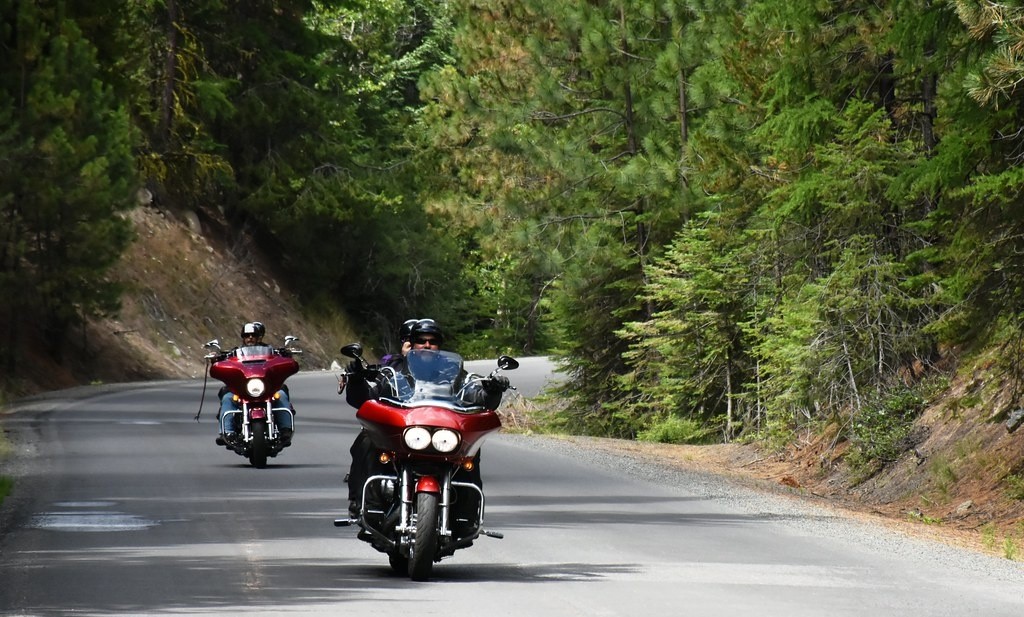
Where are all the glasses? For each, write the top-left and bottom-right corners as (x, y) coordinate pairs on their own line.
(414, 337), (440, 345)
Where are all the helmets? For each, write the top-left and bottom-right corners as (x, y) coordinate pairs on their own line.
(399, 319), (419, 342)
(410, 319), (442, 346)
(253, 322), (265, 339)
(241, 323), (258, 340)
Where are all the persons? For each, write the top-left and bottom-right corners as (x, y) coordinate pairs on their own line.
(208, 323), (293, 445)
(383, 319), (422, 377)
(217, 322), (290, 404)
(344, 319), (510, 533)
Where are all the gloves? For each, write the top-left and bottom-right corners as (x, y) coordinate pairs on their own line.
(345, 360), (365, 377)
(481, 376), (510, 394)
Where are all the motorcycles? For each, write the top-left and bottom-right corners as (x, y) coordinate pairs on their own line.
(193, 334), (303, 468)
(332, 342), (519, 582)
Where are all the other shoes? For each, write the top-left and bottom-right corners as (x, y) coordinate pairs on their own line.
(450, 517), (476, 537)
(349, 501), (366, 518)
(280, 434), (291, 447)
(216, 433), (233, 450)
